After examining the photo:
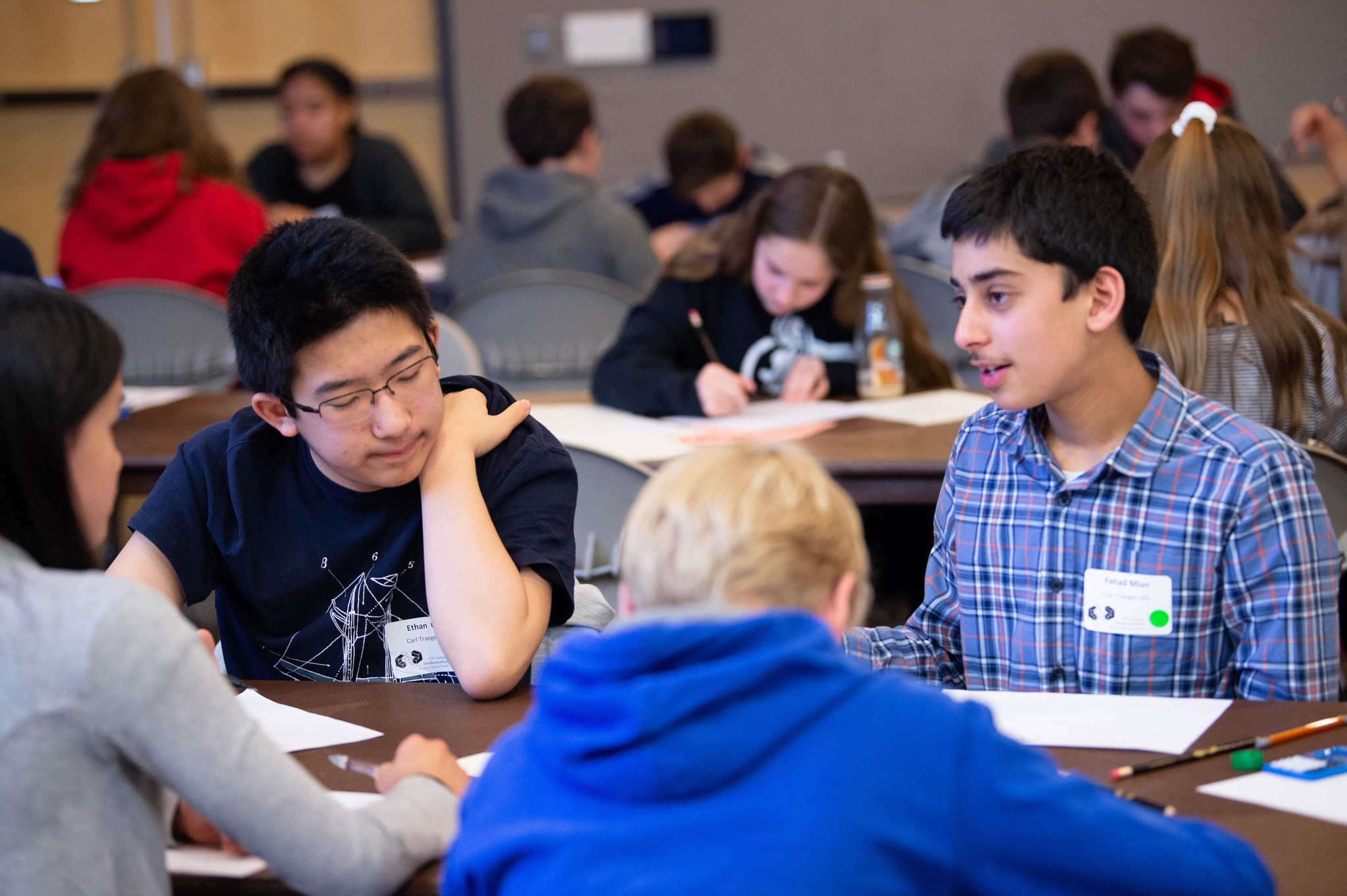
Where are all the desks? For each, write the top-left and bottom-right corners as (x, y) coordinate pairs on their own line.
(558, 387), (996, 600)
(163, 683), (1347, 896)
(105, 394), (255, 562)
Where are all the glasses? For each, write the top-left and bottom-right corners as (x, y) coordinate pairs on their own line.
(273, 324), (439, 426)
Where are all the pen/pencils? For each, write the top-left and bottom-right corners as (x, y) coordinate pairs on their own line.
(1255, 714), (1347, 749)
(1058, 770), (1176, 817)
(327, 753), (377, 778)
(1109, 737), (1257, 779)
(687, 308), (719, 362)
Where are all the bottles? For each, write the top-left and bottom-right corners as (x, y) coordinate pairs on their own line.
(855, 274), (904, 398)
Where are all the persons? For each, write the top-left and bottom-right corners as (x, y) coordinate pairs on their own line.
(820, 142), (1347, 725)
(1125, 97), (1347, 488)
(0, 222), (40, 284)
(87, 211), (583, 711)
(438, 439), (1284, 896)
(587, 164), (965, 416)
(0, 278), (489, 894)
(439, 73), (671, 314)
(1083, 25), (1238, 198)
(873, 47), (1104, 337)
(49, 65), (280, 313)
(240, 54), (452, 265)
(1280, 103), (1347, 324)
(599, 104), (795, 284)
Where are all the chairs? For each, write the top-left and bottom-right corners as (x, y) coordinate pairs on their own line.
(73, 279), (242, 386)
(432, 308), (486, 384)
(559, 442), (654, 611)
(454, 274), (646, 389)
(885, 257), (996, 400)
